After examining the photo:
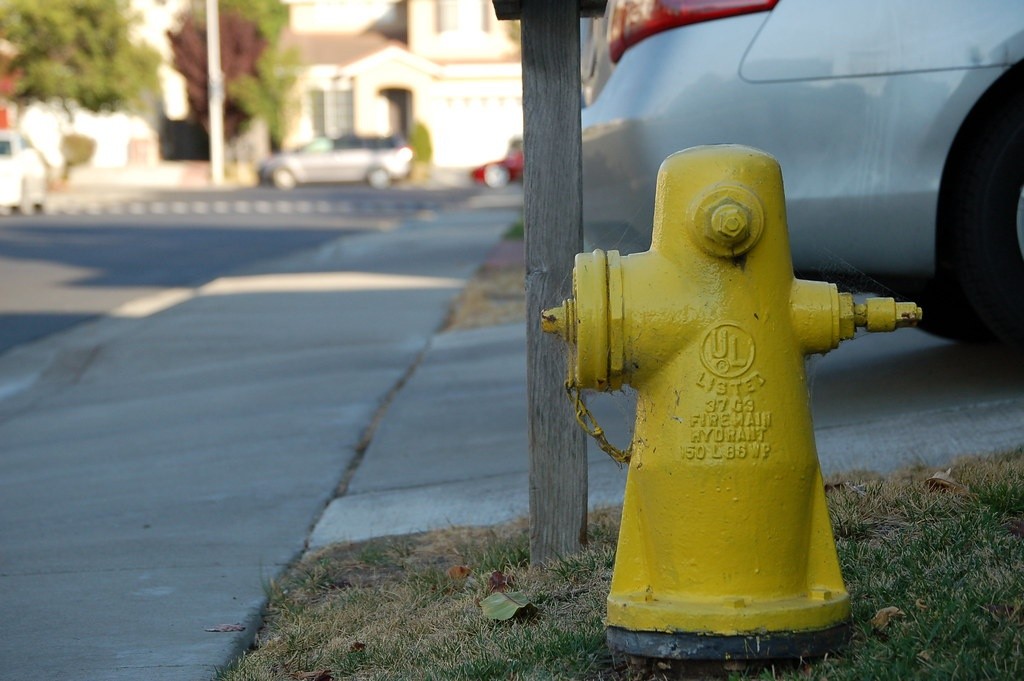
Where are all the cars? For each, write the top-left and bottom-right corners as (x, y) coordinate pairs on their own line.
(0, 129), (50, 217)
(578, 0), (1024, 353)
(468, 144), (524, 192)
(256, 131), (416, 192)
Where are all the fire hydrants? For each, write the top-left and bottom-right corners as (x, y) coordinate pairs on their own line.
(539, 140), (925, 664)
